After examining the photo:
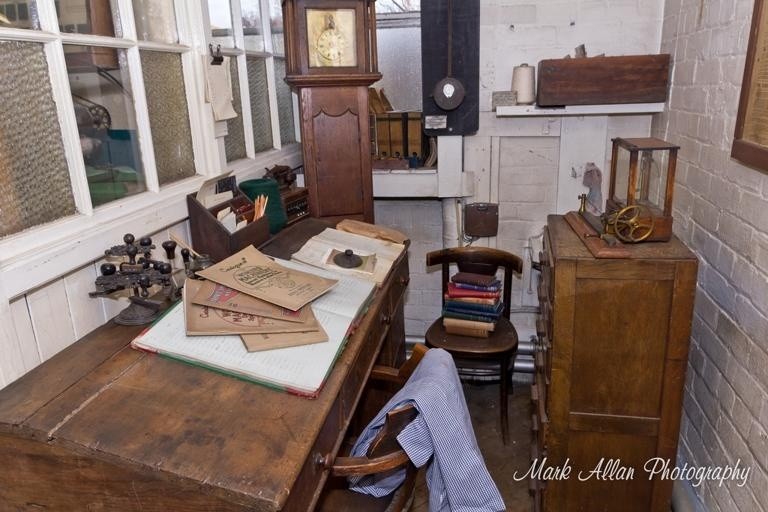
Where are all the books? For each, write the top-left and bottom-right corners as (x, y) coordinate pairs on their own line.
(441, 271), (506, 340)
(202, 53), (240, 141)
(195, 171), (269, 234)
(127, 218), (411, 400)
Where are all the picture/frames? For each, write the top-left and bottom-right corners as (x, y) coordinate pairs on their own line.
(728, 0), (767, 175)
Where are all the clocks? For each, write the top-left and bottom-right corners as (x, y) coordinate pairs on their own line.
(279, 1), (383, 225)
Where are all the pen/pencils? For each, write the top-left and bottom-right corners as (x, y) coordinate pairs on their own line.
(252, 194), (269, 222)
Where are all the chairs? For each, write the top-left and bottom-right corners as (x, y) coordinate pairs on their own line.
(317, 339), (431, 512)
(425, 247), (523, 447)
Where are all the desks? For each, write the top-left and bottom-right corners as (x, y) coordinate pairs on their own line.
(528, 213), (699, 512)
(0, 217), (414, 512)
